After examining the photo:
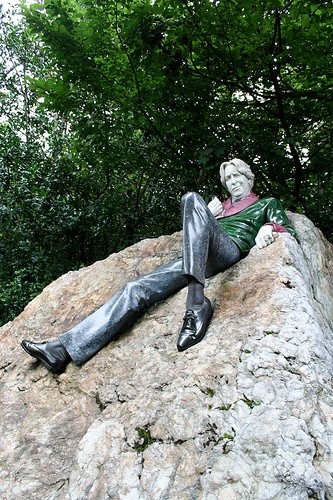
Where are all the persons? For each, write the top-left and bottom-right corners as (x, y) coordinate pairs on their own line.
(19, 158), (301, 376)
